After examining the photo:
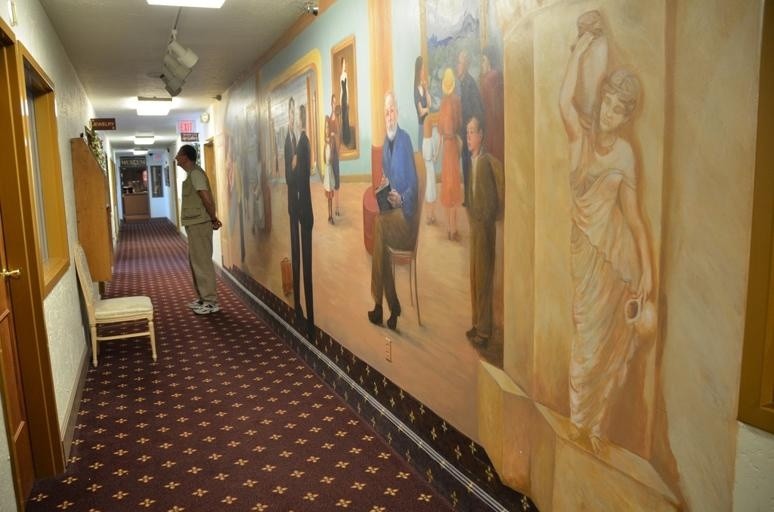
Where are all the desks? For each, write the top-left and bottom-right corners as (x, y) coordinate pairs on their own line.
(124, 191), (149, 223)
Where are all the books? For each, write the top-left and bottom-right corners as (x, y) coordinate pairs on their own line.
(375, 182), (395, 212)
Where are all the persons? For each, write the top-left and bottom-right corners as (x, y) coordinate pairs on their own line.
(412, 55), (429, 122)
(437, 68), (463, 243)
(217, 102), (274, 268)
(456, 51), (479, 209)
(174, 144), (223, 316)
(329, 95), (343, 217)
(421, 113), (439, 224)
(340, 54), (350, 144)
(463, 116), (502, 348)
(323, 115), (338, 225)
(293, 104), (315, 335)
(368, 91), (422, 331)
(479, 45), (505, 159)
(560, 10), (653, 443)
(283, 97), (303, 329)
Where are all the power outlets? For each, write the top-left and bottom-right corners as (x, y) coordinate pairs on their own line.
(384, 337), (392, 361)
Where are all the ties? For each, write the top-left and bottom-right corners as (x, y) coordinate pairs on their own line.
(291, 138), (295, 156)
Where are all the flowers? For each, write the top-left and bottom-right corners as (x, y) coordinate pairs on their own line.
(90, 137), (106, 170)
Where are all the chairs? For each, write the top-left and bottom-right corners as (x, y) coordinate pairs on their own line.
(73, 243), (157, 368)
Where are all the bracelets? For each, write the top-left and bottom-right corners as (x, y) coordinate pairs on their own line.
(212, 218), (218, 222)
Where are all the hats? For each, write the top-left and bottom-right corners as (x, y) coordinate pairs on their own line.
(441, 68), (455, 95)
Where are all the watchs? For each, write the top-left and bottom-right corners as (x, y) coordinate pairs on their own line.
(400, 194), (406, 210)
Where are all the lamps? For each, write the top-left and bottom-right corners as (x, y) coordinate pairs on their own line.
(160, 29), (198, 97)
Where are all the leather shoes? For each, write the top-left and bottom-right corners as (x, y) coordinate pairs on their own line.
(470, 336), (488, 346)
(368, 305), (384, 323)
(466, 327), (477, 337)
(387, 304), (401, 328)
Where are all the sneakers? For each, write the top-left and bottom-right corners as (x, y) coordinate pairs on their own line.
(193, 301), (221, 315)
(188, 299), (203, 309)
